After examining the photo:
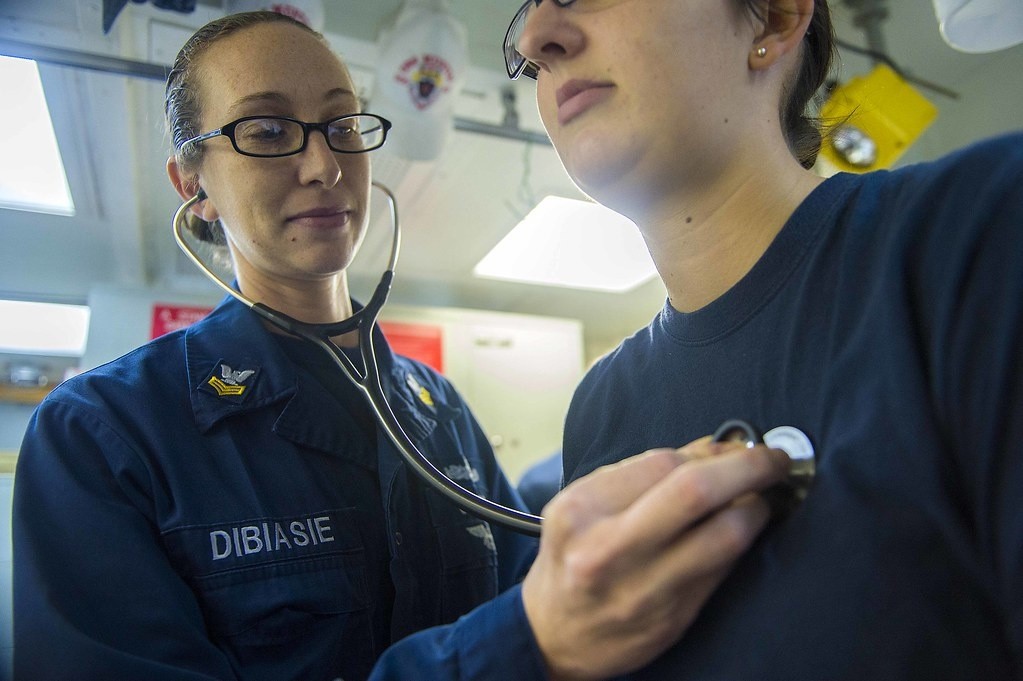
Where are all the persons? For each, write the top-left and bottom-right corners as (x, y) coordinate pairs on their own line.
(501, 1), (1023, 680)
(13, 11), (791, 680)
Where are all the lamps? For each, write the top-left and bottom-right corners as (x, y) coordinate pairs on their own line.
(816, 64), (937, 173)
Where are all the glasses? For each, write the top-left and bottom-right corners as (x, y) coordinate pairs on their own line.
(179, 113), (392, 158)
(503, 0), (578, 80)
